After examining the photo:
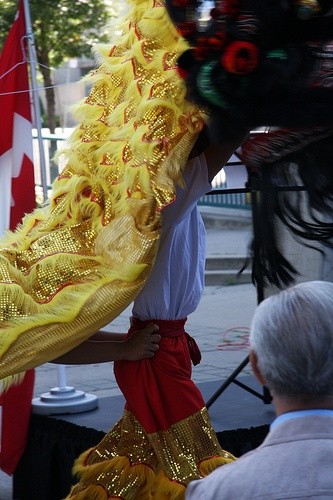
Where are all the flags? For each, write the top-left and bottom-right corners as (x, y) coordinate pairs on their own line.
(0, 2), (42, 500)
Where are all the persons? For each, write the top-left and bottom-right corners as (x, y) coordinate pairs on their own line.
(49, 318), (162, 366)
(65, 118), (239, 500)
(184, 278), (333, 500)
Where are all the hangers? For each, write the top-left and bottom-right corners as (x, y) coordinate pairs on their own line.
(0, 36), (75, 95)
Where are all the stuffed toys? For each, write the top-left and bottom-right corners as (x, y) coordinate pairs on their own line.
(0, 0), (332, 389)
